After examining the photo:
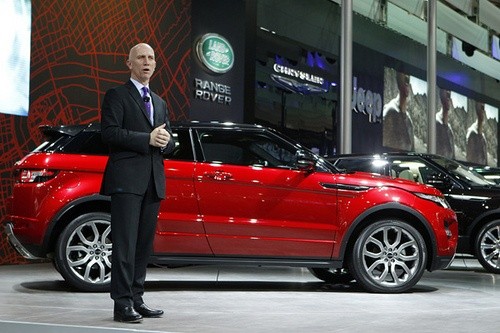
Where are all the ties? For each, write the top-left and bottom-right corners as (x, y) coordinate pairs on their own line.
(141, 86), (150, 119)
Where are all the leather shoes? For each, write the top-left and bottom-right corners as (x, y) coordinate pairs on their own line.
(136, 303), (164, 317)
(114, 307), (143, 323)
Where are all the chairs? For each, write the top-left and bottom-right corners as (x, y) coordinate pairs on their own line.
(390, 168), (416, 182)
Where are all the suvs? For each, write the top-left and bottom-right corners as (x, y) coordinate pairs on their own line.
(4, 122), (458, 294)
(321, 151), (500, 275)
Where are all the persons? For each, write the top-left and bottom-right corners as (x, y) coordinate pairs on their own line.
(436, 86), (459, 160)
(98, 42), (174, 322)
(383, 70), (416, 153)
(464, 100), (491, 167)
